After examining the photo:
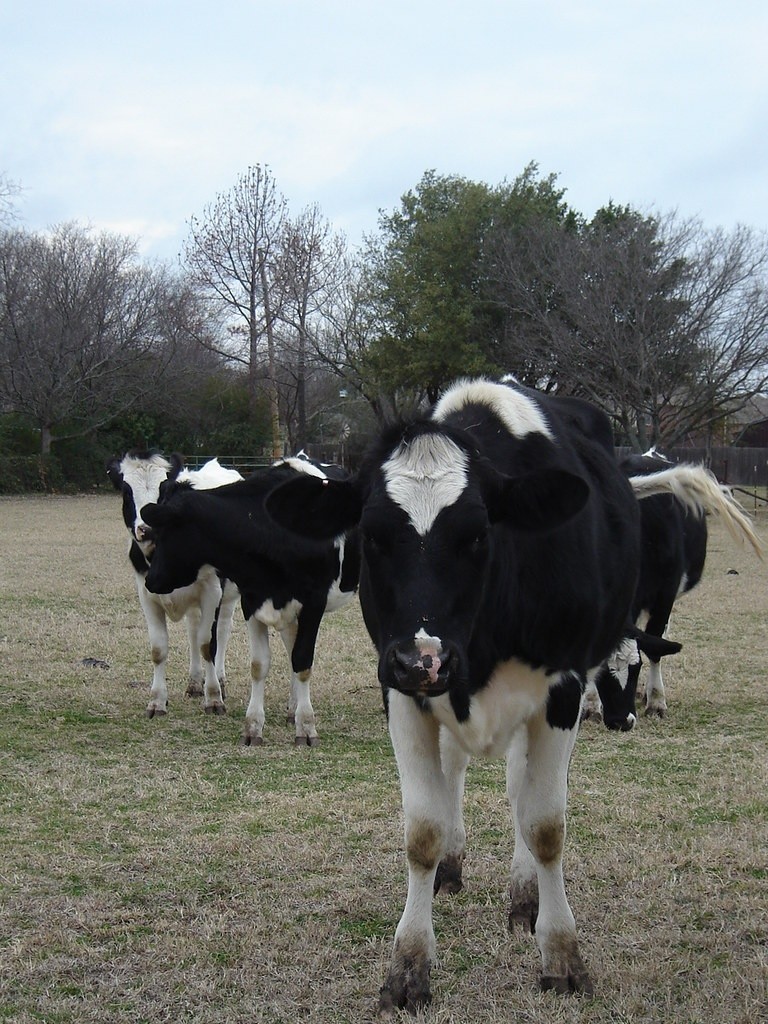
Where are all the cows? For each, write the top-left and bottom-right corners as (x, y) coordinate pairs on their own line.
(103, 370), (767, 1024)
